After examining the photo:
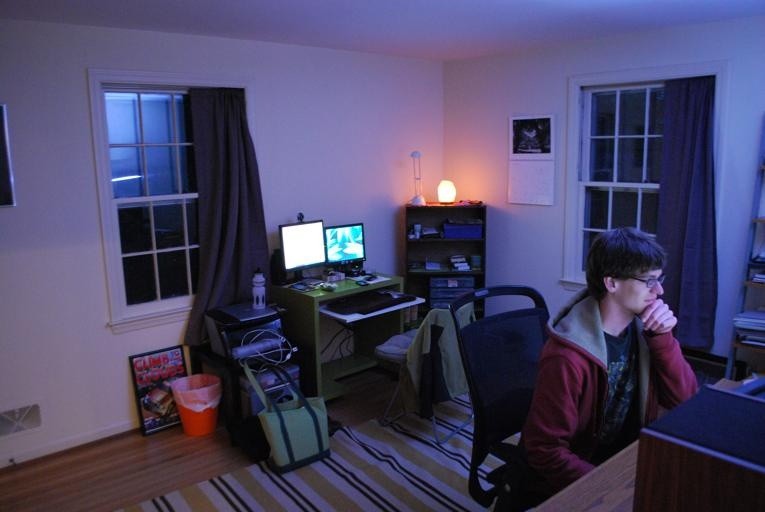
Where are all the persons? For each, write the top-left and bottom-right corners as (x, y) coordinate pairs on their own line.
(509, 227), (698, 502)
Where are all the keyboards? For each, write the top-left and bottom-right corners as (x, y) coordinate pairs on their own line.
(327, 292), (392, 314)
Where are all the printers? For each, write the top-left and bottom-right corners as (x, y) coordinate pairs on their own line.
(204, 301), (284, 360)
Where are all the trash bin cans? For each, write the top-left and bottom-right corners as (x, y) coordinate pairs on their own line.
(171, 373), (223, 435)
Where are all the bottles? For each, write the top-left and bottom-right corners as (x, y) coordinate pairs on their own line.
(252, 267), (266, 310)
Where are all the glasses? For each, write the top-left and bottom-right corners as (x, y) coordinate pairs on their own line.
(621, 273), (667, 288)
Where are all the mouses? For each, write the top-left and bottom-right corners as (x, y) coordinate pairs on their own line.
(387, 292), (400, 299)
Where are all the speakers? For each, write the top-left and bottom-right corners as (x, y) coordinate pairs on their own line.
(633, 384), (765, 512)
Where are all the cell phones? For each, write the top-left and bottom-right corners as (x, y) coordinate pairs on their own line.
(356, 281), (369, 286)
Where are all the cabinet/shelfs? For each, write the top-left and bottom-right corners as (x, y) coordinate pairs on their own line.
(723, 110), (764, 381)
(400, 201), (487, 335)
(190, 343), (301, 448)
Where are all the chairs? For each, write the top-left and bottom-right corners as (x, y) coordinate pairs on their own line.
(375, 302), (477, 445)
(449, 284), (583, 511)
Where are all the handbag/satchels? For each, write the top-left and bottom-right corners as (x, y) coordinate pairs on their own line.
(243, 357), (331, 474)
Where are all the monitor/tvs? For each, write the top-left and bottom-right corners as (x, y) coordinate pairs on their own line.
(279, 220), (327, 284)
(324, 223), (365, 277)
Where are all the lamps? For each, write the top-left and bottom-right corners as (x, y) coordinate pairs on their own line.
(409, 151), (426, 207)
(438, 180), (456, 203)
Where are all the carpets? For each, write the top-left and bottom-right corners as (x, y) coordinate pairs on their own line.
(113, 389), (522, 512)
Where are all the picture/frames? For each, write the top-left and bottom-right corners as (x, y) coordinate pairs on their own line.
(129, 345), (188, 437)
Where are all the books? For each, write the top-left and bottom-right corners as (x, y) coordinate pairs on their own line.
(733, 309), (765, 348)
(751, 277), (765, 283)
(753, 270), (765, 278)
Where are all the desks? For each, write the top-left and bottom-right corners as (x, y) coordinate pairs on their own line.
(272, 271), (427, 405)
(526, 379), (744, 511)
